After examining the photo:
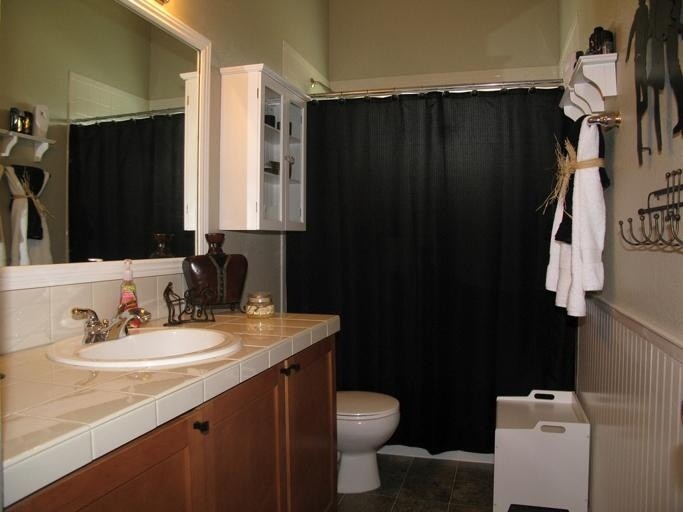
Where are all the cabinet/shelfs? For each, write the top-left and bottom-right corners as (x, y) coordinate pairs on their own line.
(219, 63), (313, 232)
(204, 338), (335, 510)
(6, 407), (209, 511)
(491, 389), (590, 511)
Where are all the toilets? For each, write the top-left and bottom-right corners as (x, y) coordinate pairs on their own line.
(333, 388), (403, 497)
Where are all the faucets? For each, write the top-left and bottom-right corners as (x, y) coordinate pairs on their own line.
(69, 303), (151, 342)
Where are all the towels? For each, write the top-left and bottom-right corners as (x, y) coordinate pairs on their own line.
(3, 165), (54, 266)
(540, 113), (609, 320)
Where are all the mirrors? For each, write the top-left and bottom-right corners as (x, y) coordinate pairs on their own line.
(0, 0), (214, 295)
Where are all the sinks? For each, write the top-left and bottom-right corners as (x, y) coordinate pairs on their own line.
(47, 322), (244, 370)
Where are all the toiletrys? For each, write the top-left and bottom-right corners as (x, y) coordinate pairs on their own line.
(115, 256), (142, 328)
